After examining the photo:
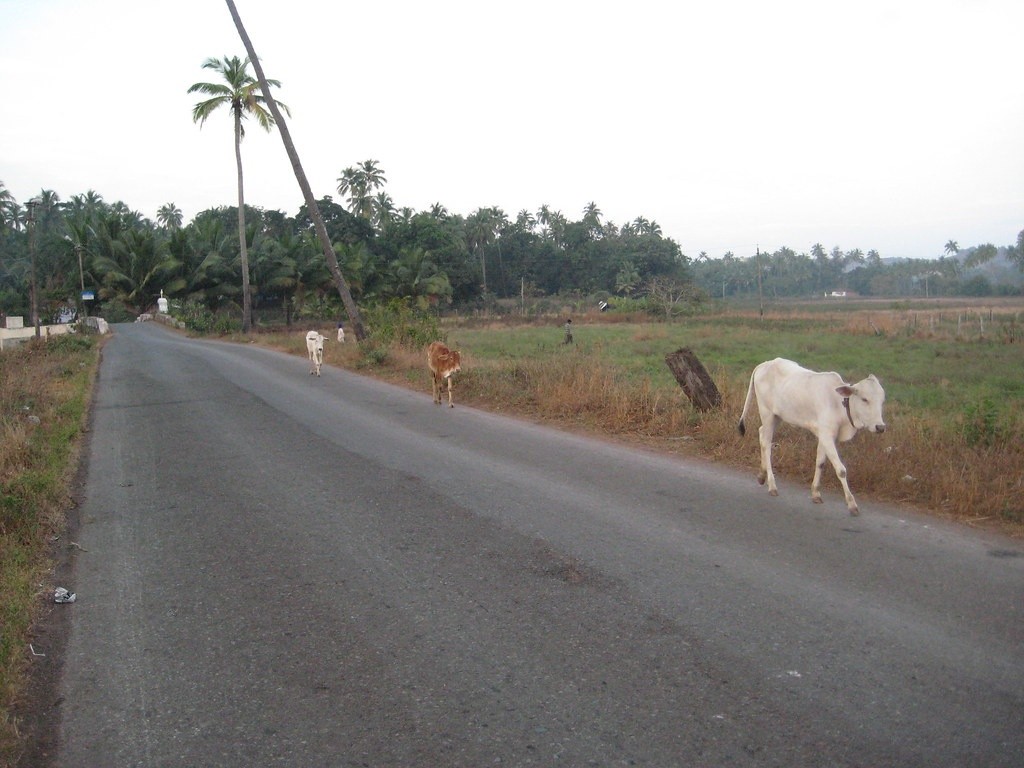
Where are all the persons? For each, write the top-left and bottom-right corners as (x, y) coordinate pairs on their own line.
(336, 323), (344, 343)
(563, 319), (572, 345)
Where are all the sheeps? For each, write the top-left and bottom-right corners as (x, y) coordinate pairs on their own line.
(740, 356), (887, 515)
(305, 331), (330, 376)
(428, 341), (462, 409)
